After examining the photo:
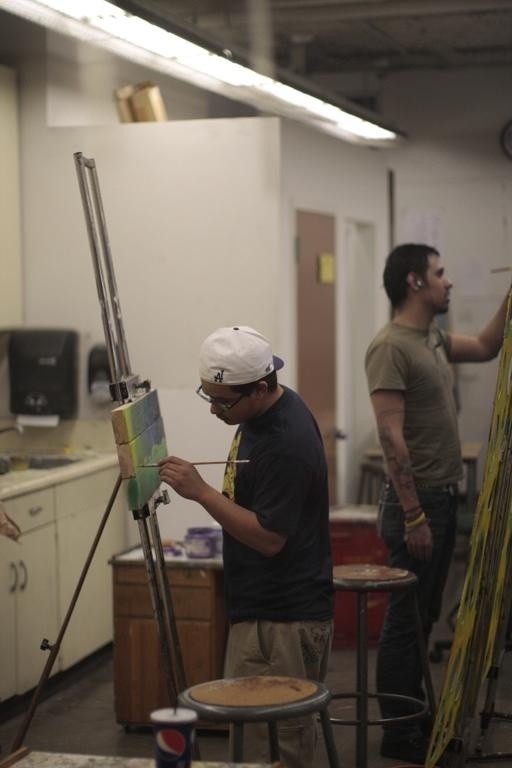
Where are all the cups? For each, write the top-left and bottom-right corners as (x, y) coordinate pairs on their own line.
(149, 708), (197, 768)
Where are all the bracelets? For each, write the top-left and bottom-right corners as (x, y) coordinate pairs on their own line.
(402, 505), (426, 528)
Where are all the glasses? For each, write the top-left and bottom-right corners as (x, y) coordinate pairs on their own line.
(196, 384), (245, 411)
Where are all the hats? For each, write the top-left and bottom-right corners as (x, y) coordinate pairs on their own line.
(196, 326), (284, 384)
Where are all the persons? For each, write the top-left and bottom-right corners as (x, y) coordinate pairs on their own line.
(158, 326), (335, 768)
(364, 244), (512, 762)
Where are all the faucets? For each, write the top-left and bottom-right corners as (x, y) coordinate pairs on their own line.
(0, 422), (24, 436)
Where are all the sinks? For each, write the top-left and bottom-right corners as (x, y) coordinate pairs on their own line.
(4, 450), (80, 473)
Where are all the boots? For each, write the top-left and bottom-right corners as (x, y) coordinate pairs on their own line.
(379, 716), (463, 765)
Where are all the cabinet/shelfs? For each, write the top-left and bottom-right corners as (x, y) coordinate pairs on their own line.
(56, 466), (129, 681)
(105, 538), (229, 736)
(0, 487), (61, 714)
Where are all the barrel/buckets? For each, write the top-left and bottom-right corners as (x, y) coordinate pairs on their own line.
(329, 504), (392, 651)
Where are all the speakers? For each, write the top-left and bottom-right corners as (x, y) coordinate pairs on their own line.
(9, 329), (78, 417)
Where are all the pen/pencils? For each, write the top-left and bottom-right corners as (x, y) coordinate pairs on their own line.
(490, 266), (512, 274)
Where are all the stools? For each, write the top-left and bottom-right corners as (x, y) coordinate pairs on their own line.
(177, 675), (341, 767)
(314, 563), (437, 767)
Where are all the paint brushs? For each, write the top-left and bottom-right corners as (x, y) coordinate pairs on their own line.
(137, 460), (250, 467)
